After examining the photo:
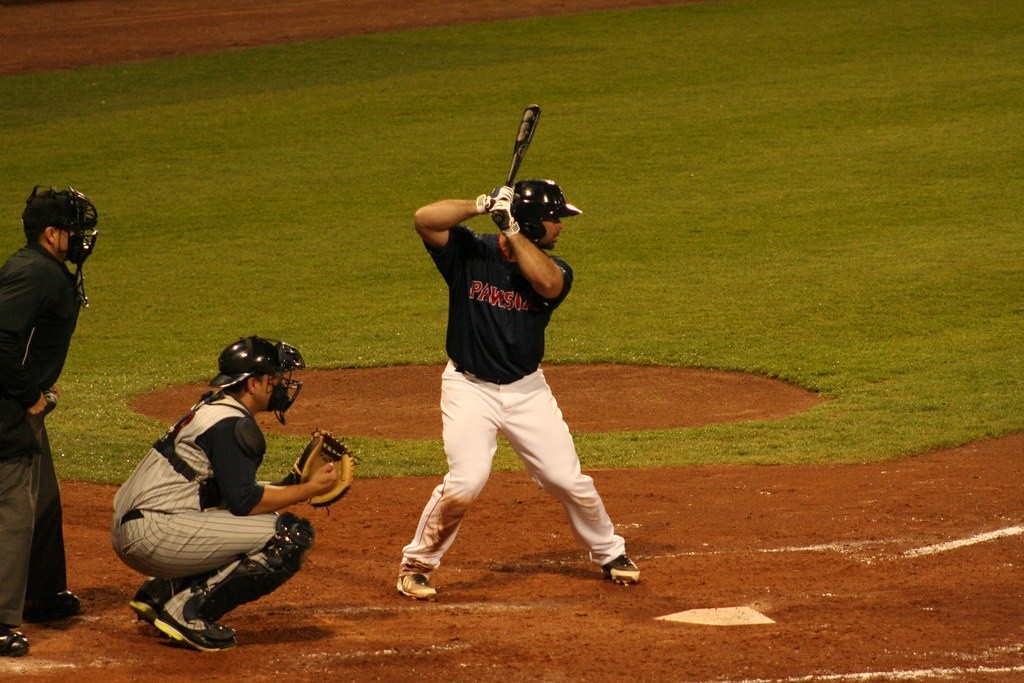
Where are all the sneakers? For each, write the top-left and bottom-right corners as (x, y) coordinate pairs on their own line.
(130, 589), (161, 627)
(601, 556), (640, 586)
(153, 608), (237, 651)
(397, 574), (436, 601)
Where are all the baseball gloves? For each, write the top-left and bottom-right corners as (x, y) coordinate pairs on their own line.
(294, 426), (354, 508)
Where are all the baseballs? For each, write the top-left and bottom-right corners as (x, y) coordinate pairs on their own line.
(45, 392), (58, 404)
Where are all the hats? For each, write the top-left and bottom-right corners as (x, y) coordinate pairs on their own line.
(24, 191), (97, 231)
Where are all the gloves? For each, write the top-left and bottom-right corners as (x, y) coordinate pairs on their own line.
(476, 186), (521, 237)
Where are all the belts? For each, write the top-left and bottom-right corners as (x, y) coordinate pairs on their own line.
(475, 374), (523, 385)
(116, 509), (144, 530)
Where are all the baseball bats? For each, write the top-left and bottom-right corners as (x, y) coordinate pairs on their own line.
(492, 105), (542, 229)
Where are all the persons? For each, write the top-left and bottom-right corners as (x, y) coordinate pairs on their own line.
(112, 335), (353, 653)
(396, 179), (641, 601)
(0, 187), (97, 657)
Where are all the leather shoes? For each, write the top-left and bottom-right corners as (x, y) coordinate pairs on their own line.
(23, 589), (80, 617)
(0, 629), (29, 657)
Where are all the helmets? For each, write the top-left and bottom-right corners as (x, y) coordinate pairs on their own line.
(511, 179), (582, 243)
(208, 336), (281, 388)
